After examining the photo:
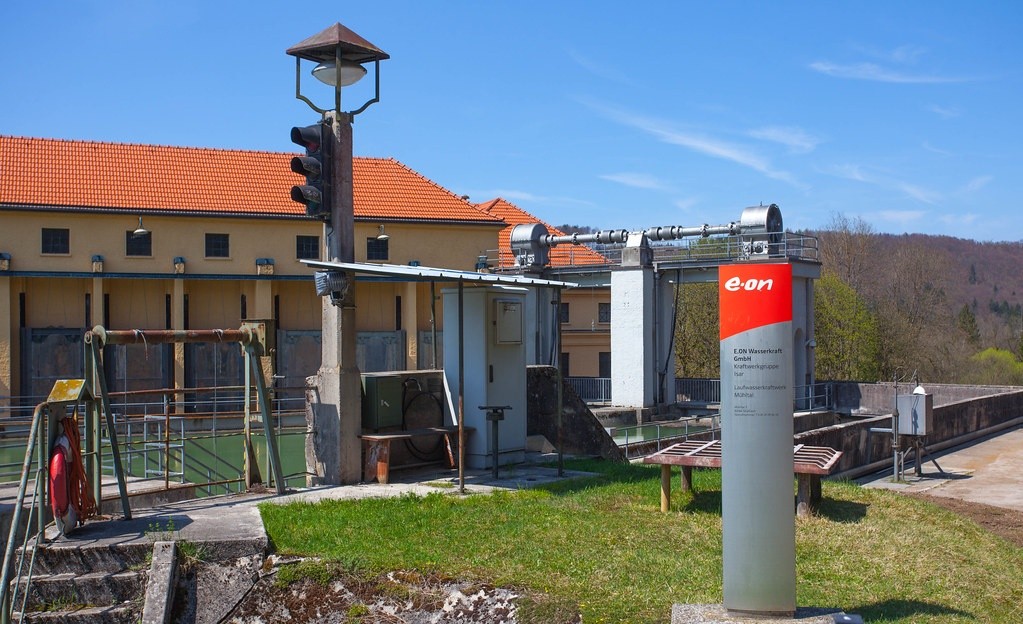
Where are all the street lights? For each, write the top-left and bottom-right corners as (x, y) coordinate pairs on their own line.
(285, 23), (391, 487)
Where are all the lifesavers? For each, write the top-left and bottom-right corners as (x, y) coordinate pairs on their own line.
(52, 414), (83, 537)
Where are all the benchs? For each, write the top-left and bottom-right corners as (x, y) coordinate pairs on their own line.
(356, 426), (476, 484)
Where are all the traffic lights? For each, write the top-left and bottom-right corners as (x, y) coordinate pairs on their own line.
(289, 123), (336, 220)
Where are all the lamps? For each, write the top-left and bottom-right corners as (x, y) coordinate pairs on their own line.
(377, 225), (389, 240)
(133, 217), (149, 235)
